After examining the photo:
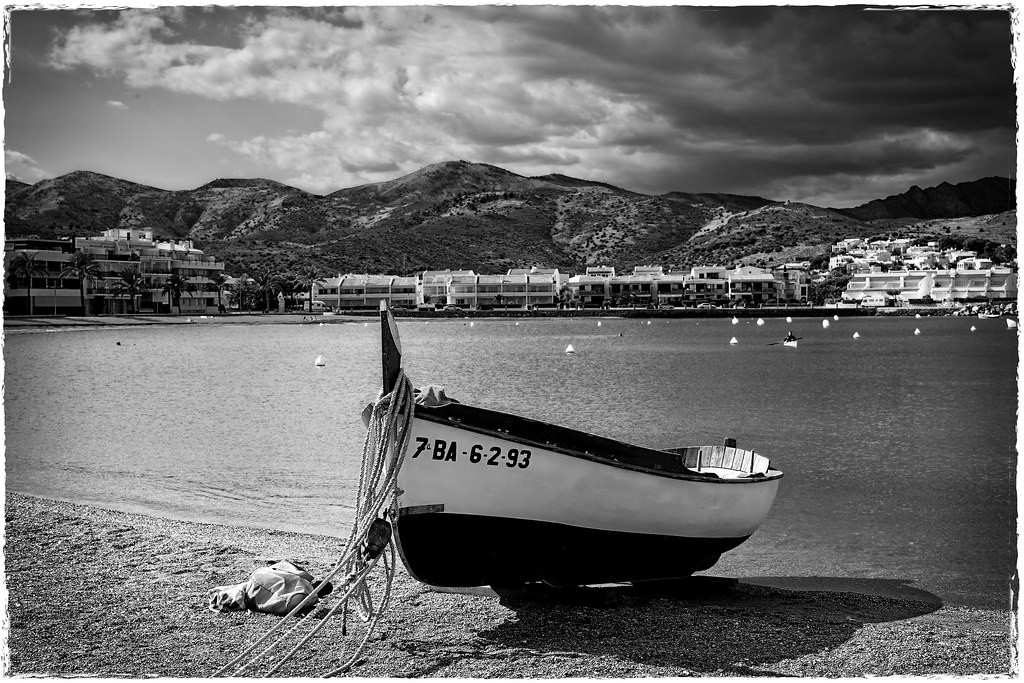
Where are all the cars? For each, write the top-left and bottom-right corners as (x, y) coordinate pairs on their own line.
(443, 305), (462, 312)
(697, 303), (715, 309)
(658, 303), (674, 310)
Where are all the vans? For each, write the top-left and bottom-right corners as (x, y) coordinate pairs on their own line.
(303, 300), (330, 311)
(860, 296), (889, 307)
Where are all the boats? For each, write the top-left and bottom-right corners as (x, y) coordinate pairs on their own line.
(784, 340), (799, 349)
(360, 296), (786, 591)
(978, 313), (1000, 320)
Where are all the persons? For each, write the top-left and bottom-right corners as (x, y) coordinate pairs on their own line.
(784, 331), (796, 342)
